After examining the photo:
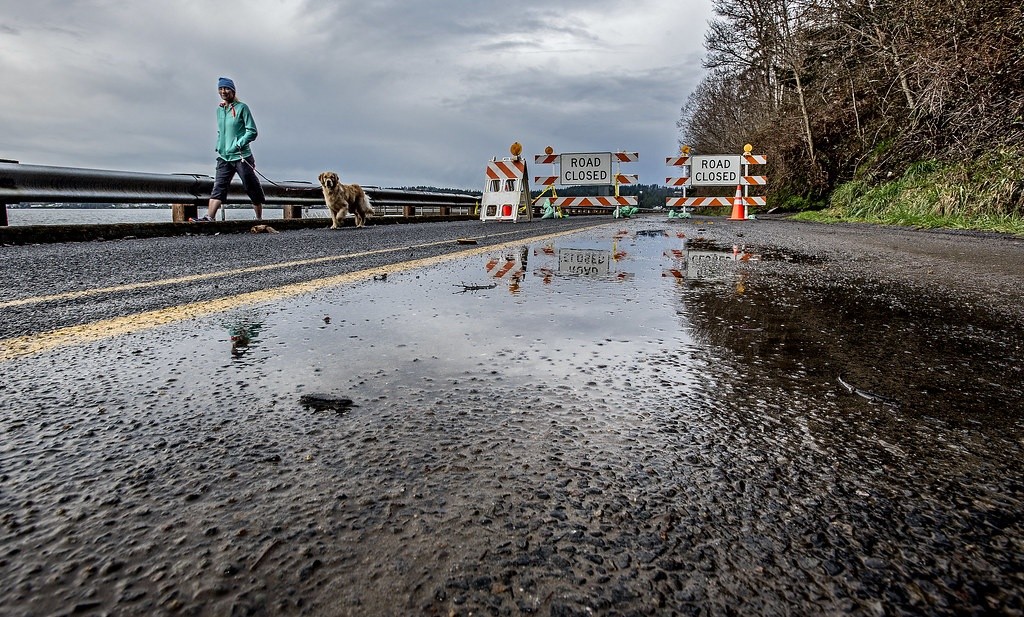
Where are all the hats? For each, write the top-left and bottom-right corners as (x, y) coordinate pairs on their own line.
(217, 78), (236, 94)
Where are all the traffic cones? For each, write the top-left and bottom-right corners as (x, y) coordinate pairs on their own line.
(725, 184), (749, 221)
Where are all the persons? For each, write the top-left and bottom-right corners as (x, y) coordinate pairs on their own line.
(189, 77), (266, 222)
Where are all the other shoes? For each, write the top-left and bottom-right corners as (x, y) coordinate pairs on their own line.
(190, 216), (215, 222)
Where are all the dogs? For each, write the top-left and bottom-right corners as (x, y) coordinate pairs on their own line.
(319, 171), (375, 230)
